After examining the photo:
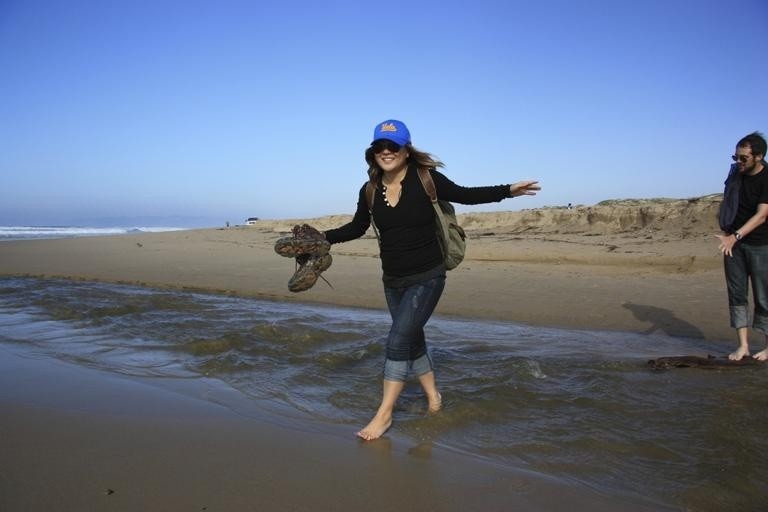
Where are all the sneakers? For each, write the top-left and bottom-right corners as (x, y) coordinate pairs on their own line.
(287, 253), (332, 292)
(274, 224), (330, 256)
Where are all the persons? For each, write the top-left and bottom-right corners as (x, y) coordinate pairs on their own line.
(295, 119), (542, 440)
(714, 131), (768, 361)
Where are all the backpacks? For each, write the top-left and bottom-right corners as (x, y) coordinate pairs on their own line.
(365, 167), (465, 270)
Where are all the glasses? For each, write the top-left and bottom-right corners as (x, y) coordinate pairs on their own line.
(371, 141), (400, 154)
(731, 155), (752, 162)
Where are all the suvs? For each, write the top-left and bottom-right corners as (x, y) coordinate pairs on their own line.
(246, 217), (258, 225)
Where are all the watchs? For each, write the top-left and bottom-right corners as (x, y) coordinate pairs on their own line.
(733, 231), (742, 242)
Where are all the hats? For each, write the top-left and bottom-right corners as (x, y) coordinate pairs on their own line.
(370, 119), (410, 147)
